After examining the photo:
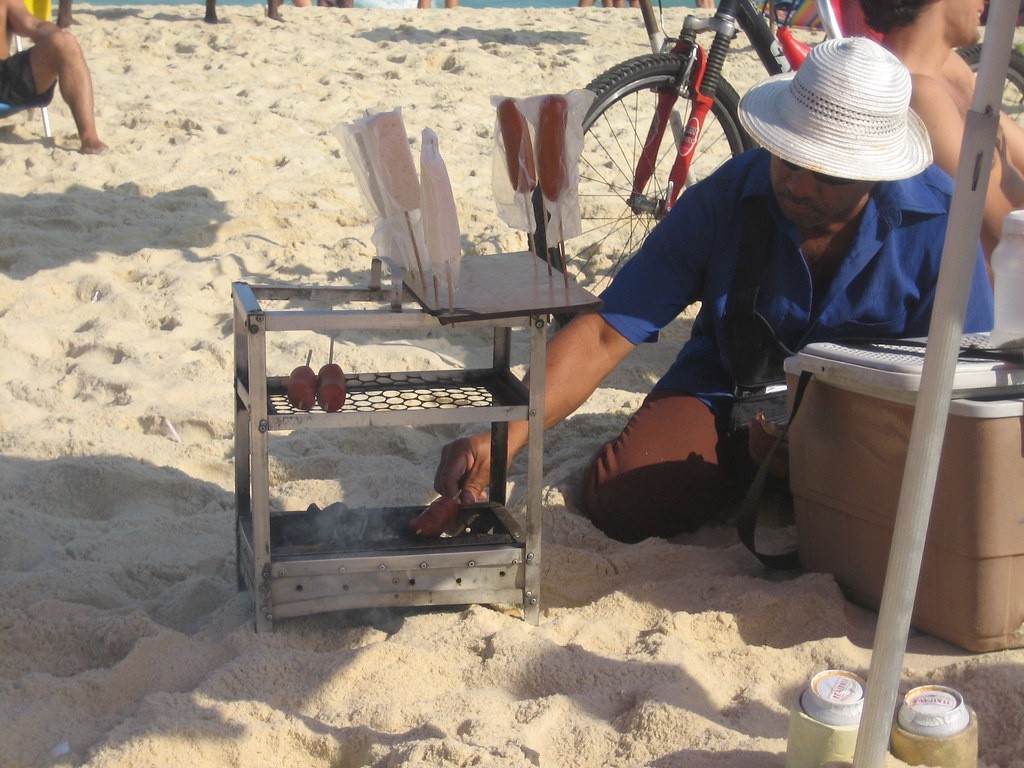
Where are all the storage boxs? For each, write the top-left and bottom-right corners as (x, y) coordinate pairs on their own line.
(784, 331), (1024, 653)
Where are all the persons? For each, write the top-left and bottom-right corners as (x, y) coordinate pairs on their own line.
(204, 0), (459, 24)
(578, 0), (715, 9)
(434, 38), (994, 543)
(859, 1), (1024, 293)
(0, 0), (108, 155)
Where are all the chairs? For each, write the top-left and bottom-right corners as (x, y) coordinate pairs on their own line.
(0, 0), (53, 138)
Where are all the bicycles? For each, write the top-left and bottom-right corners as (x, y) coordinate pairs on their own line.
(528, 0), (1024, 327)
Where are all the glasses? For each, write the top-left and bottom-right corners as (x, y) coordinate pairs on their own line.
(780, 158), (855, 184)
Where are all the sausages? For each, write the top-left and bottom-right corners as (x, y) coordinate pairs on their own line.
(287, 363), (346, 412)
(407, 497), (458, 539)
(497, 94), (569, 201)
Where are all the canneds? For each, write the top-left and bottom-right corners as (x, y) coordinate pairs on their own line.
(800, 669), (869, 725)
(897, 684), (970, 737)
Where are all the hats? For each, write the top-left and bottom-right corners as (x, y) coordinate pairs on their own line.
(737, 35), (934, 182)
